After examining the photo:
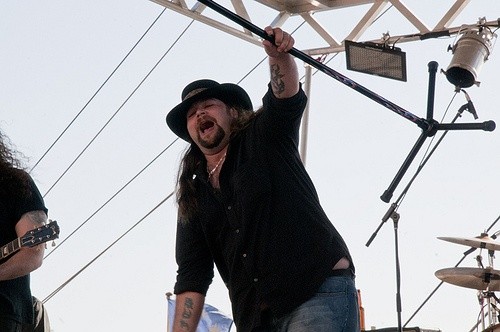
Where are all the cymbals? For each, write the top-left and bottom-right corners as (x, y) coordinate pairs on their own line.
(435, 266), (500, 292)
(436, 234), (500, 251)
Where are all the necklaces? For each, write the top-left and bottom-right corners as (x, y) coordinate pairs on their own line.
(206, 153), (227, 178)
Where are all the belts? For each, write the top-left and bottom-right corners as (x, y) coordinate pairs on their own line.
(329, 269), (351, 276)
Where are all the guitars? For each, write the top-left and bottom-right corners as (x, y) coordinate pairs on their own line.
(0, 219), (61, 264)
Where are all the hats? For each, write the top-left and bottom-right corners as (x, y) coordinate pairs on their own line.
(166, 79), (253, 144)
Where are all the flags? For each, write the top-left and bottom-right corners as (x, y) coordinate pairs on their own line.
(167, 300), (234, 332)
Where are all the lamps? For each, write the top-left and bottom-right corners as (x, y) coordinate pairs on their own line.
(344, 33), (407, 82)
(445, 26), (496, 88)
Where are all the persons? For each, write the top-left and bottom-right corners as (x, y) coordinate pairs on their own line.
(164, 26), (363, 332)
(0, 131), (51, 332)
